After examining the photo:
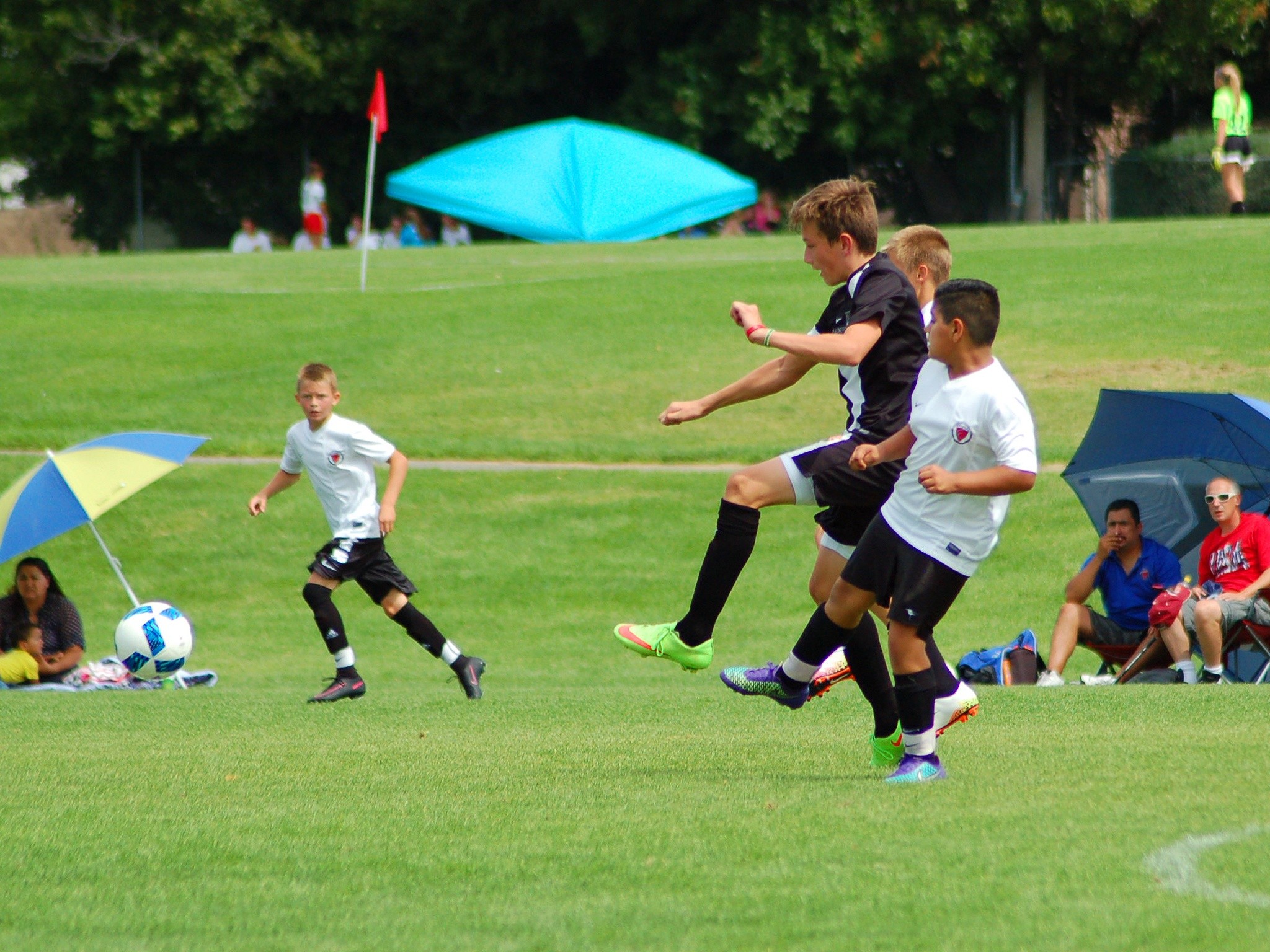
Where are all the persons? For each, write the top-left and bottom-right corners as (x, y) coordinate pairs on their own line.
(0, 557), (85, 684)
(1210, 61), (1253, 213)
(807, 223), (979, 737)
(249, 364), (485, 702)
(1034, 498), (1183, 687)
(613, 172), (928, 766)
(1159, 476), (1270, 684)
(231, 218), (272, 253)
(720, 275), (1037, 778)
(440, 215), (473, 246)
(0, 623), (64, 689)
(299, 163), (332, 251)
(293, 205), (436, 249)
(677, 190), (780, 238)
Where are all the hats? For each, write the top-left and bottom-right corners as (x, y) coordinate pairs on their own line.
(1147, 583), (1191, 631)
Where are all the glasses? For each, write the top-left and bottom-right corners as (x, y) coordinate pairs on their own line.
(1204, 493), (1238, 504)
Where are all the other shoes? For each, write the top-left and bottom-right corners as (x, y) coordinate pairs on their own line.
(1201, 669), (1221, 684)
(1174, 669), (1189, 685)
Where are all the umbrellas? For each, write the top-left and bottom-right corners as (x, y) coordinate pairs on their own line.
(0, 433), (213, 691)
(1060, 387), (1270, 660)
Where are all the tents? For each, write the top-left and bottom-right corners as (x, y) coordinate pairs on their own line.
(386, 117), (756, 246)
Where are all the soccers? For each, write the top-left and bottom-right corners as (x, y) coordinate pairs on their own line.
(114, 602), (192, 680)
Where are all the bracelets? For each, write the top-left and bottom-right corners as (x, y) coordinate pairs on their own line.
(764, 330), (774, 346)
(747, 325), (764, 339)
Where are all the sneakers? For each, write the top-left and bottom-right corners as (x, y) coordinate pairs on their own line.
(613, 619), (714, 673)
(308, 675), (366, 703)
(1080, 674), (1113, 686)
(446, 657), (486, 700)
(1034, 670), (1063, 687)
(869, 720), (905, 769)
(882, 752), (946, 785)
(806, 645), (856, 703)
(720, 662), (810, 711)
(932, 680), (980, 739)
